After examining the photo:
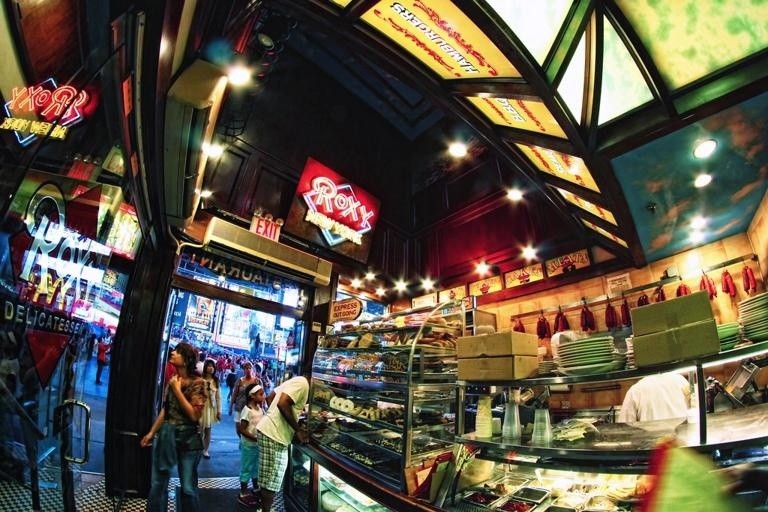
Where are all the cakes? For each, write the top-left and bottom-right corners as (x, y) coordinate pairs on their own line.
(320, 482), (358, 512)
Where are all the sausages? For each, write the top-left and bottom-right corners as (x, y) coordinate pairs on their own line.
(512, 314), (570, 337)
(699, 264), (757, 299)
(579, 301), (631, 331)
(638, 282), (689, 304)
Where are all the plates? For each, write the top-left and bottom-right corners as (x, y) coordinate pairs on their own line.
(736, 291), (768, 343)
(536, 359), (555, 377)
(714, 322), (743, 352)
(554, 335), (628, 376)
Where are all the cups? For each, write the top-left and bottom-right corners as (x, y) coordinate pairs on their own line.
(530, 409), (553, 445)
(502, 403), (523, 439)
(474, 399), (492, 439)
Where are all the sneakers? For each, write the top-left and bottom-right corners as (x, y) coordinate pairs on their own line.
(252, 489), (266, 508)
(238, 491), (257, 507)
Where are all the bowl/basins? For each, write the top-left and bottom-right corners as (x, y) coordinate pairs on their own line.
(320, 491), (359, 512)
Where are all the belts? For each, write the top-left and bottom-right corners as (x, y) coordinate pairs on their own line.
(162, 418), (197, 425)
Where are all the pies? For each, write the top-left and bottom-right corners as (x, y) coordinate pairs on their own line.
(418, 330), (460, 348)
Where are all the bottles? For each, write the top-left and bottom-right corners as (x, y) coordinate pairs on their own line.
(294, 474), (309, 501)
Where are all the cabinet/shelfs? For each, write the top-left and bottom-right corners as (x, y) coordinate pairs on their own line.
(456, 337), (767, 460)
(307, 299), (467, 493)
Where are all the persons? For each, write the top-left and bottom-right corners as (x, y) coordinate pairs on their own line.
(238, 384), (266, 500)
(139, 342), (208, 511)
(615, 374), (691, 427)
(161, 337), (282, 439)
(75, 328), (113, 385)
(254, 369), (310, 511)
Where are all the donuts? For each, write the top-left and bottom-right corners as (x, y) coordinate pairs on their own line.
(330, 397), (402, 421)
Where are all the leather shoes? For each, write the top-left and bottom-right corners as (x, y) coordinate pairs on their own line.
(203, 448), (210, 459)
(96, 381), (103, 385)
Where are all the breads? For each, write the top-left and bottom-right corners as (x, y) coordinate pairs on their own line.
(322, 320), (408, 348)
(326, 353), (409, 372)
